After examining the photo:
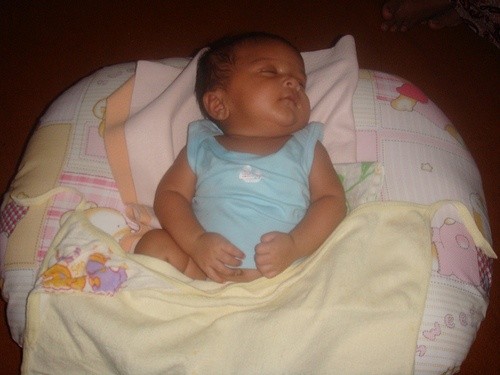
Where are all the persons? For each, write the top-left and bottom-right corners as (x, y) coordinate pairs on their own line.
(134, 28), (349, 286)
